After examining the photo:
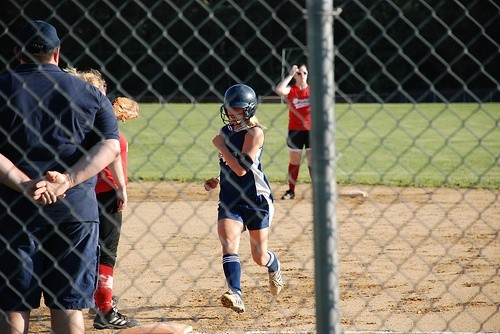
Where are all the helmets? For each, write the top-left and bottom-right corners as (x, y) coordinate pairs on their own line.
(224, 84), (258, 119)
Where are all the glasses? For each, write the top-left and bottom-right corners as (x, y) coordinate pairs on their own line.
(297, 72), (307, 75)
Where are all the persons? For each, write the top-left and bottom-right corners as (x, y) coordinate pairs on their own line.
(63, 66), (140, 329)
(277, 59), (313, 199)
(204, 85), (282, 312)
(0, 21), (121, 334)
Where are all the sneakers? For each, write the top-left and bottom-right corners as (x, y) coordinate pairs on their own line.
(93, 307), (140, 329)
(268, 259), (282, 296)
(221, 289), (246, 313)
(87, 296), (120, 316)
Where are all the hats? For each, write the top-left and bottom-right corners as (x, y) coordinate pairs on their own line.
(19, 18), (62, 53)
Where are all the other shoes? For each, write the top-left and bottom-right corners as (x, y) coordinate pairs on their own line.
(281, 190), (295, 200)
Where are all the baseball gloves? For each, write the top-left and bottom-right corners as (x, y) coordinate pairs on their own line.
(111, 97), (139, 123)
